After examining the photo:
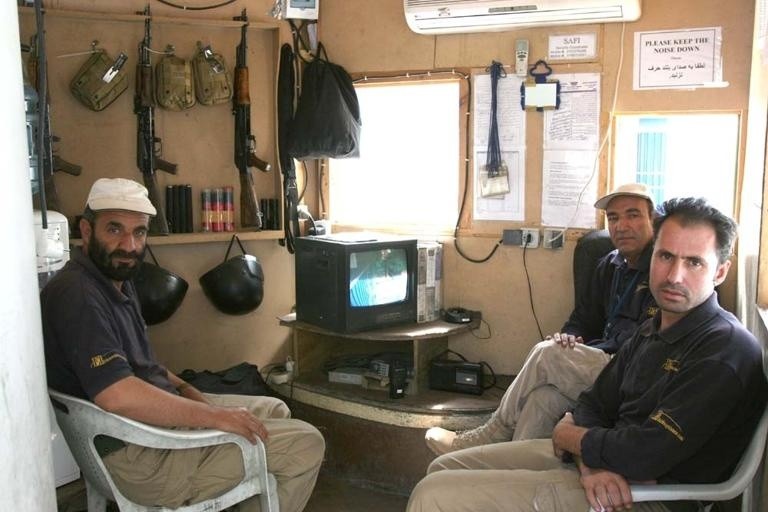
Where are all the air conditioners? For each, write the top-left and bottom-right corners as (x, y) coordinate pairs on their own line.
(404, 0), (641, 36)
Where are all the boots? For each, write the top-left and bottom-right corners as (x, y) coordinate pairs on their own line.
(424, 412), (514, 456)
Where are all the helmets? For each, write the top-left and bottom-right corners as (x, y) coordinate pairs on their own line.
(130, 260), (189, 324)
(199, 254), (265, 316)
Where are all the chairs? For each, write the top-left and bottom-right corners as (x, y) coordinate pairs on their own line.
(47, 390), (280, 512)
(589, 411), (768, 512)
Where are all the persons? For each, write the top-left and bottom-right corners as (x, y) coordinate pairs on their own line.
(425, 183), (664, 456)
(40, 176), (328, 512)
(404, 200), (768, 512)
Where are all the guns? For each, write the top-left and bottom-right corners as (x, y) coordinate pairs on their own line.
(232, 7), (271, 228)
(21, 1), (82, 238)
(135, 3), (178, 235)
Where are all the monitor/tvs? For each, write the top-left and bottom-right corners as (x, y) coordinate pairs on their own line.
(296, 229), (418, 334)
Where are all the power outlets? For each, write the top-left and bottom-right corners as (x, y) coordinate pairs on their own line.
(520, 228), (540, 248)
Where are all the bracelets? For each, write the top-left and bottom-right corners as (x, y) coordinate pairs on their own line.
(177, 380), (194, 389)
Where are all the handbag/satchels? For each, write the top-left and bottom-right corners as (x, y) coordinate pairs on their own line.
(294, 41), (362, 161)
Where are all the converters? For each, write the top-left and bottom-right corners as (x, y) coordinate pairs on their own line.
(543, 232), (563, 247)
(501, 228), (522, 246)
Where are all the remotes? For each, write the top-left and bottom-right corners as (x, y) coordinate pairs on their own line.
(513, 38), (528, 76)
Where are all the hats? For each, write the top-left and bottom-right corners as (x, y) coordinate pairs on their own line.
(84, 177), (157, 217)
(594, 183), (656, 210)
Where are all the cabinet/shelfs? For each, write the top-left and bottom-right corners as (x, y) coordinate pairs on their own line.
(17, 1), (293, 247)
(275, 307), (516, 428)
